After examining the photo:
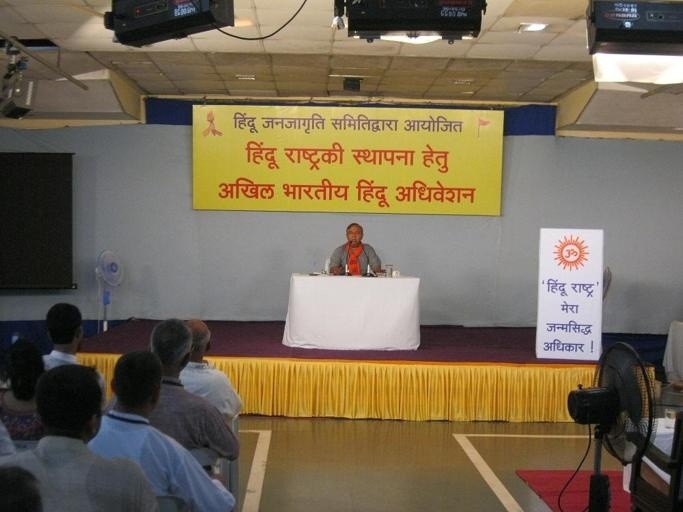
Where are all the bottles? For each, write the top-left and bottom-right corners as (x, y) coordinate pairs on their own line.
(385, 264), (401, 278)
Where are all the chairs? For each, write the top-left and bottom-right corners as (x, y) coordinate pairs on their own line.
(630, 411), (683, 512)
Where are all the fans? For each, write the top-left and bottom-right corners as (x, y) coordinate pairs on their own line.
(565, 341), (655, 512)
(93, 248), (124, 334)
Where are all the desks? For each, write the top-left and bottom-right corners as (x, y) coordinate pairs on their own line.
(662, 318), (683, 388)
(281, 273), (420, 356)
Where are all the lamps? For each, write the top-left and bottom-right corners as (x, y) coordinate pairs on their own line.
(0, 33), (41, 121)
(101, 1), (236, 54)
(331, 0), (487, 47)
(585, 0), (683, 88)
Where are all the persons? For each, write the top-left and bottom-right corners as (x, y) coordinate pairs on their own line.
(80, 349), (237, 511)
(109, 318), (240, 467)
(0, 302), (158, 511)
(329, 223), (384, 277)
(178, 319), (245, 426)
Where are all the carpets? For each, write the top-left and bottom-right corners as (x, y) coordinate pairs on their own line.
(513, 465), (631, 511)
